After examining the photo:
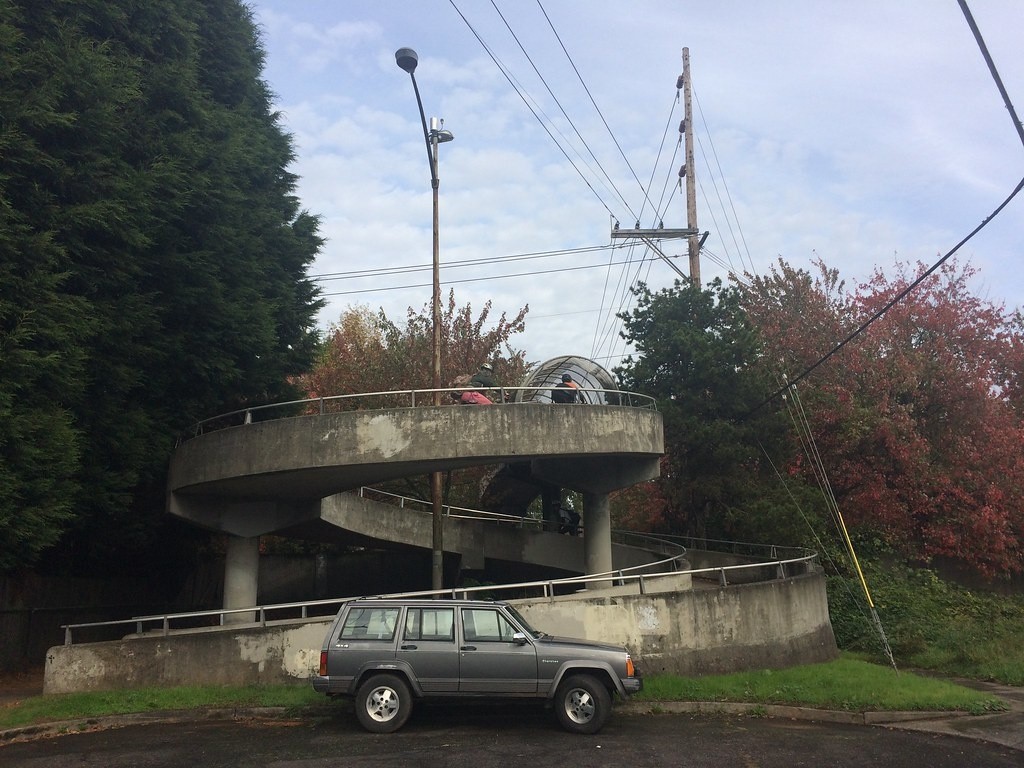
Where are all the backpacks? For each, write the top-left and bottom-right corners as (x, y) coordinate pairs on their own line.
(561, 508), (580, 525)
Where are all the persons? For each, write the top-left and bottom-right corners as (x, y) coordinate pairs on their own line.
(551, 374), (587, 404)
(460, 363), (511, 405)
(553, 502), (579, 536)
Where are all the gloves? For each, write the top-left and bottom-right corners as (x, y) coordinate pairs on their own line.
(505, 390), (510, 399)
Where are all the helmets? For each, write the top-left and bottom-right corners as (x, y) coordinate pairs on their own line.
(481, 363), (493, 371)
(552, 500), (561, 505)
(562, 374), (572, 382)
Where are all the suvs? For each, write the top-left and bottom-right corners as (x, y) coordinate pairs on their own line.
(311, 594), (643, 734)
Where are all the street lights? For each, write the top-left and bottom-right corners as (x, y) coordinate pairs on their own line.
(393, 47), (456, 600)
